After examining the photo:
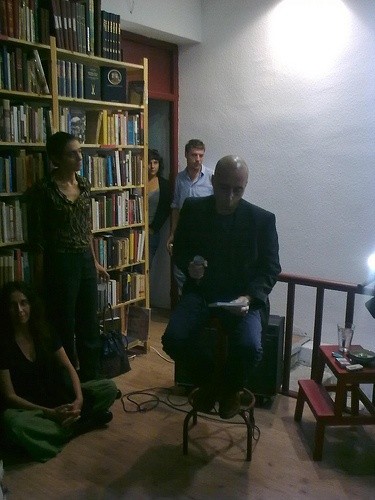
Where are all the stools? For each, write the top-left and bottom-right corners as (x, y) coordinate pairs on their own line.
(183, 318), (257, 461)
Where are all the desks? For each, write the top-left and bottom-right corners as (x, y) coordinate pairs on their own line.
(316, 345), (375, 416)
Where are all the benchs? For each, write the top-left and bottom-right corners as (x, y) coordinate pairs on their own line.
(295, 380), (336, 461)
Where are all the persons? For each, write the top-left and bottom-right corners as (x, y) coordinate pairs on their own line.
(166, 139), (215, 310)
(161, 155), (282, 420)
(148, 149), (174, 270)
(28, 132), (110, 381)
(0, 281), (118, 464)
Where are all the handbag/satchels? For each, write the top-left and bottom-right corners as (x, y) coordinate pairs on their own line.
(99, 304), (131, 379)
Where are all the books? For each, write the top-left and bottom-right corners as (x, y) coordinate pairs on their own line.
(0, 0), (151, 346)
(208, 302), (246, 307)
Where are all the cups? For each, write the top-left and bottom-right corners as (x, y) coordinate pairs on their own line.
(97, 272), (107, 292)
(169, 243), (173, 256)
(337, 322), (355, 353)
(191, 256), (204, 279)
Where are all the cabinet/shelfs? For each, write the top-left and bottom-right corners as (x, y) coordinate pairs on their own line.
(1, 34), (148, 353)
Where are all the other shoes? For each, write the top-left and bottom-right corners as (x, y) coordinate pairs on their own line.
(79, 411), (112, 432)
(195, 382), (217, 411)
(218, 391), (241, 418)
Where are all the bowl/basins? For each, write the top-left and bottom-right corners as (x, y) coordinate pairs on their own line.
(348, 349), (375, 364)
